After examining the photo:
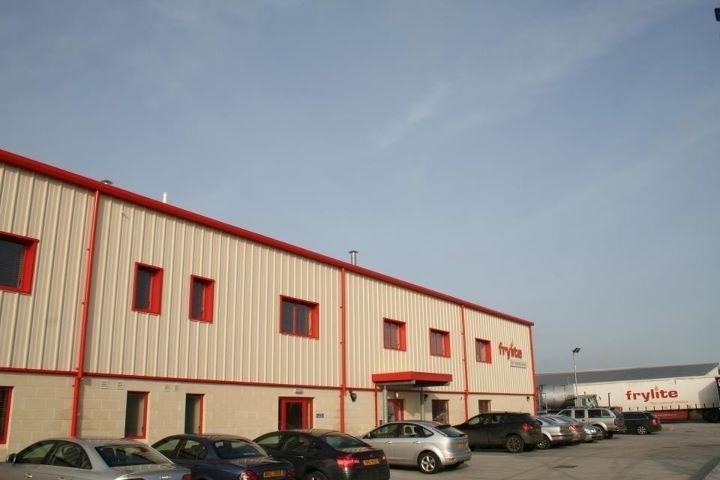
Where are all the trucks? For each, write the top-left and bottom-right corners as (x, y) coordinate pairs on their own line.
(560, 376), (720, 424)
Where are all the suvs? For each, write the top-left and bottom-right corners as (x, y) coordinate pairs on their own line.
(450, 411), (543, 454)
(554, 406), (619, 440)
(621, 413), (663, 435)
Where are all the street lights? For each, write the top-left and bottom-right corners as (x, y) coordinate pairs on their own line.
(572, 347), (581, 396)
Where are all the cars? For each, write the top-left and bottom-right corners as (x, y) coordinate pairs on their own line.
(355, 419), (473, 474)
(0, 437), (193, 480)
(532, 414), (603, 449)
(238, 430), (391, 480)
(149, 433), (297, 480)
